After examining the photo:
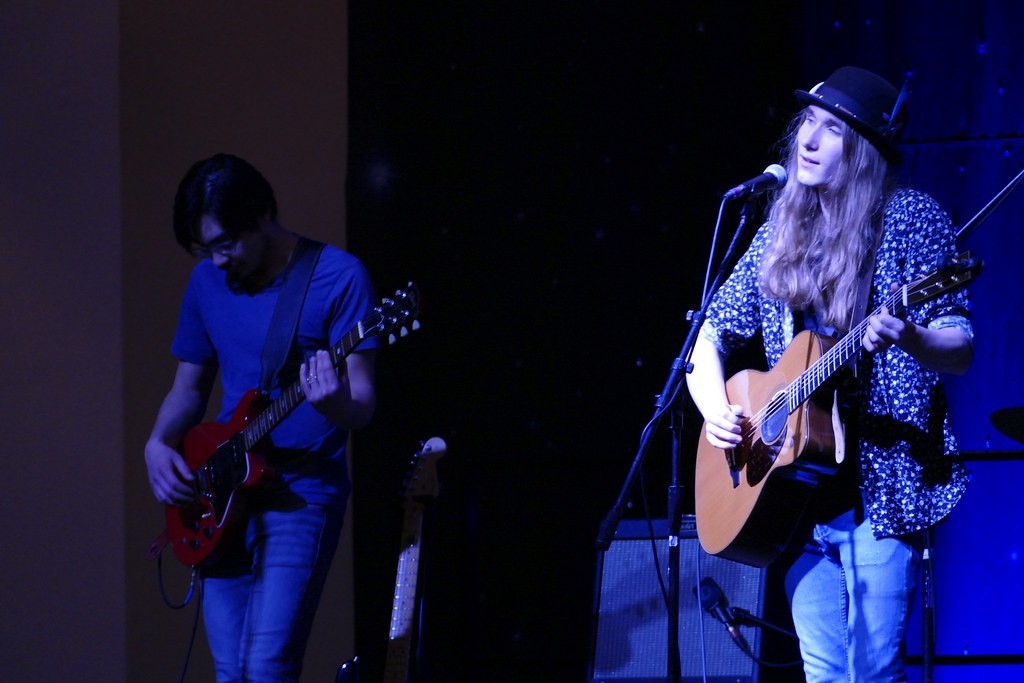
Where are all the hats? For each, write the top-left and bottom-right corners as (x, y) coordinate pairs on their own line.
(794, 66), (915, 167)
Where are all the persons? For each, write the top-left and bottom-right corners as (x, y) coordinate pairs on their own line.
(144, 152), (381, 683)
(685, 67), (975, 683)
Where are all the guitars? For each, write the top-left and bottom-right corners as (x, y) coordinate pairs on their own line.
(167, 278), (430, 569)
(334, 433), (451, 683)
(693, 242), (980, 567)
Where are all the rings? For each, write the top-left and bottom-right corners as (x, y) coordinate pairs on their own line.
(306, 376), (318, 384)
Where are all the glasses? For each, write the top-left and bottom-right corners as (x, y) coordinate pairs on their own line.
(189, 240), (237, 259)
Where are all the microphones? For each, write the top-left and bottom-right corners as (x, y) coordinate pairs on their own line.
(723, 165), (787, 201)
(693, 576), (750, 654)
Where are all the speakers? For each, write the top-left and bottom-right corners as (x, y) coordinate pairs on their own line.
(591, 515), (807, 683)
